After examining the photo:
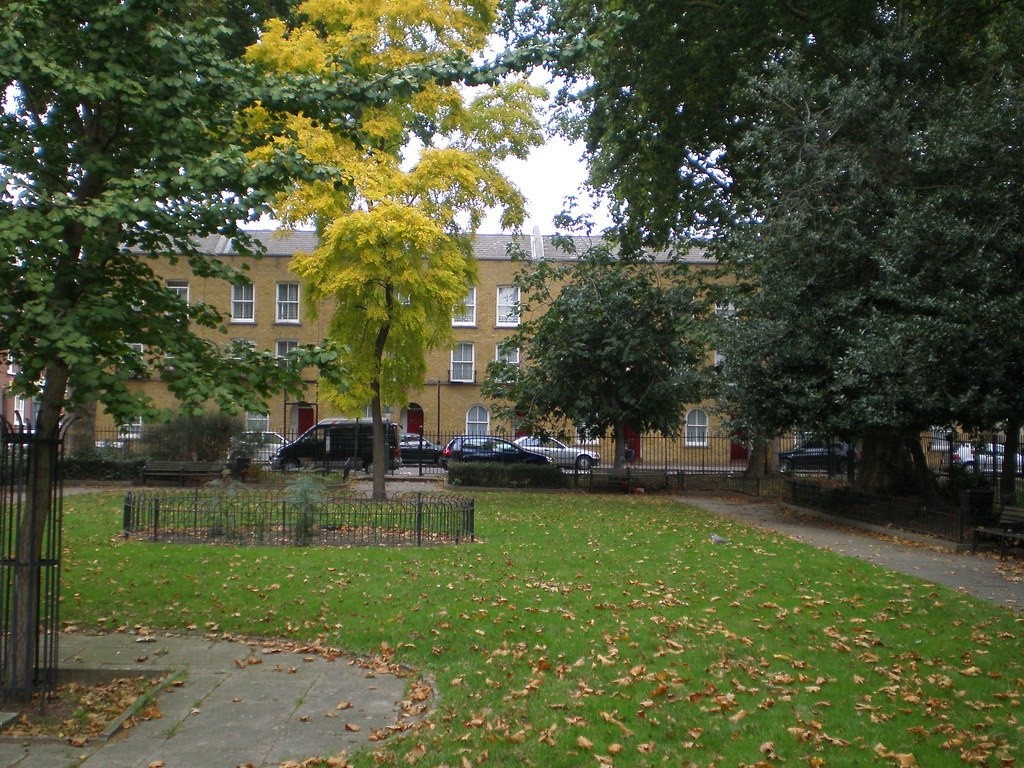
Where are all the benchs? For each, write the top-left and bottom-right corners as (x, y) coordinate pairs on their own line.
(141, 460), (225, 487)
(972, 505), (1024, 561)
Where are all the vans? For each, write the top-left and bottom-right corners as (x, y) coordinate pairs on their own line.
(270, 416), (402, 474)
(226, 430), (291, 461)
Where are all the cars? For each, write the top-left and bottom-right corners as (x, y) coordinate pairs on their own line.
(400, 433), (446, 467)
(496, 436), (601, 470)
(779, 439), (861, 474)
(941, 442), (1022, 476)
(439, 436), (556, 471)
